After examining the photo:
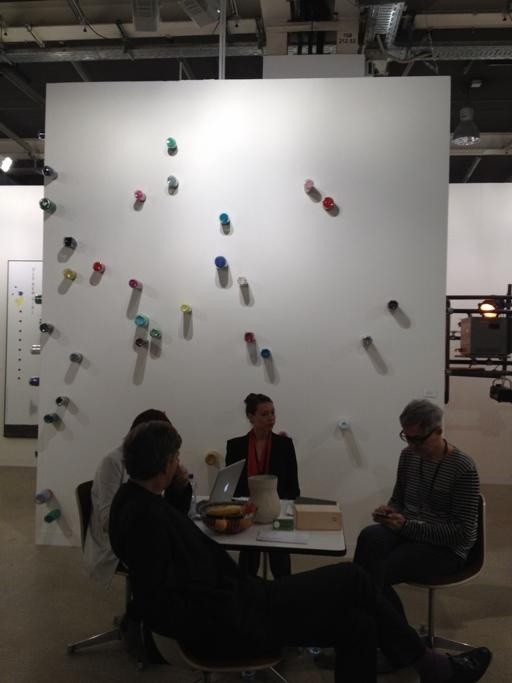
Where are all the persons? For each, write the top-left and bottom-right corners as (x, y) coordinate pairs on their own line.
(109, 419), (490, 683)
(78, 408), (173, 591)
(314, 399), (480, 671)
(225, 390), (302, 580)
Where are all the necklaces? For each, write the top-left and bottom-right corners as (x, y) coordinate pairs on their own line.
(253, 428), (270, 475)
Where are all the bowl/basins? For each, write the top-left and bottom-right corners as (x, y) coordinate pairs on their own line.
(199, 502), (257, 534)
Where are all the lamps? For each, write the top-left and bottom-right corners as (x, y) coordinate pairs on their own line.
(476, 292), (510, 320)
(452, 105), (482, 144)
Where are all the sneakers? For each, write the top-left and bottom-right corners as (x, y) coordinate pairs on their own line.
(371, 646), (397, 673)
(314, 647), (336, 669)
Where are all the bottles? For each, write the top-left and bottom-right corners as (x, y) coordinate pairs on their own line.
(187, 474), (199, 519)
(284, 501), (296, 529)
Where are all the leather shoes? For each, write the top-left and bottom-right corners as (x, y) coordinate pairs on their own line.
(421, 646), (492, 682)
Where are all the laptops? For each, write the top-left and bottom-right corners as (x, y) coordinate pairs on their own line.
(196, 459), (246, 520)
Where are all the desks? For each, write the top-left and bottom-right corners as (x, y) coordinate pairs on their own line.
(179, 488), (350, 579)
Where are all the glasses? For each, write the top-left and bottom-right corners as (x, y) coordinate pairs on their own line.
(399, 427), (440, 445)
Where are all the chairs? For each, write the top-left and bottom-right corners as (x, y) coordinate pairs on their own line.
(401, 492), (489, 652)
(119, 548), (297, 683)
(66, 473), (157, 671)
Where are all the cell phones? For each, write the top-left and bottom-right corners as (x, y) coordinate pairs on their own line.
(376, 512), (388, 518)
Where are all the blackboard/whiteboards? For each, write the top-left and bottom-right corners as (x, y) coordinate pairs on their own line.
(36, 76), (448, 547)
